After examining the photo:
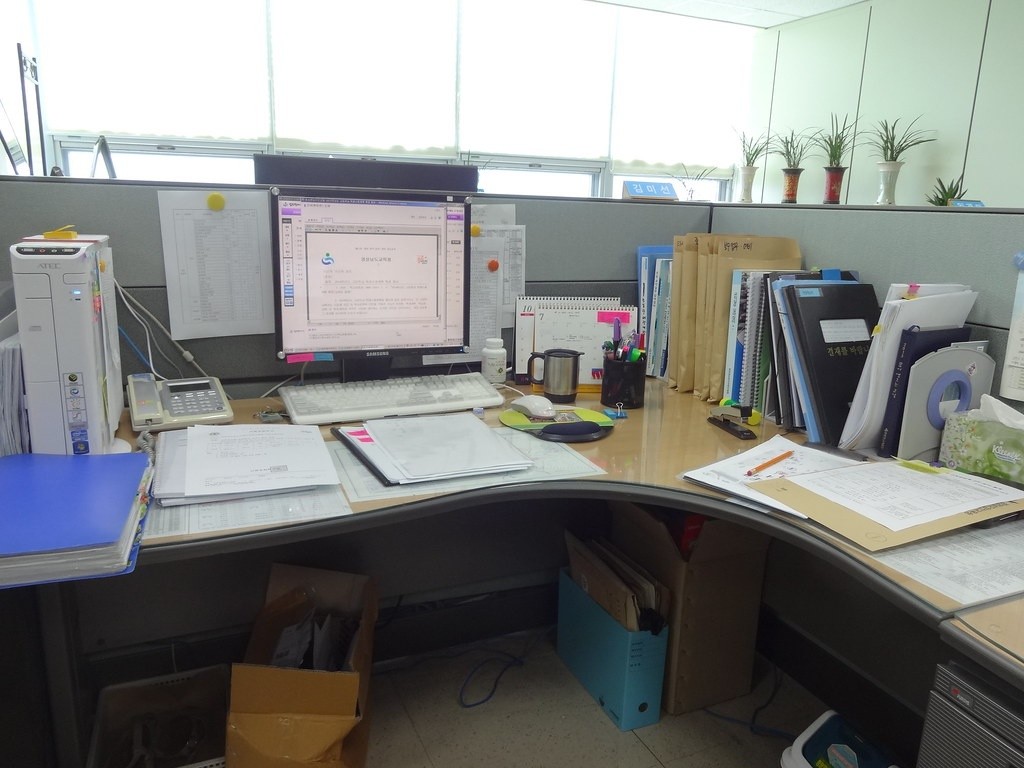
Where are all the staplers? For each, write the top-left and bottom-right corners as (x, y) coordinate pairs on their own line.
(707, 404), (758, 441)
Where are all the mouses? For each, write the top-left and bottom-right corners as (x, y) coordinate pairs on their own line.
(510, 394), (556, 420)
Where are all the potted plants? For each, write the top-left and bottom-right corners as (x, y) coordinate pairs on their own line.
(861, 113), (938, 204)
(738, 131), (779, 203)
(810, 113), (868, 204)
(765, 127), (823, 202)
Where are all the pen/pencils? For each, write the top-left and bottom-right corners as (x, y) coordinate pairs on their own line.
(747, 450), (794, 477)
(600, 317), (647, 361)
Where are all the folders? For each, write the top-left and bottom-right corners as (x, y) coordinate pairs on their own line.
(0, 452), (155, 590)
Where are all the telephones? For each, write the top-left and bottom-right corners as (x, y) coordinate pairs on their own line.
(126, 373), (233, 432)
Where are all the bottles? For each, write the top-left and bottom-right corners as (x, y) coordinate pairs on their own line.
(481, 338), (507, 382)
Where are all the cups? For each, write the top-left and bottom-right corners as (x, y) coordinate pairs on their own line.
(527, 349), (585, 404)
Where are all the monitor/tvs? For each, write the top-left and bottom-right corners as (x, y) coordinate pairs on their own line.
(269, 186), (470, 383)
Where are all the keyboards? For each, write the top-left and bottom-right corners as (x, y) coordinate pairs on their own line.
(278, 372), (503, 425)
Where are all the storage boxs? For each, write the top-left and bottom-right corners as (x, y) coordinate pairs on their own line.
(611, 500), (771, 715)
(226, 561), (379, 767)
(86, 663), (232, 768)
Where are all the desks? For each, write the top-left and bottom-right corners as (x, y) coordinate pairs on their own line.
(119, 375), (1023, 694)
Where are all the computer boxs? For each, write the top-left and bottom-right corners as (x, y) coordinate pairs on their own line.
(9, 233), (124, 455)
